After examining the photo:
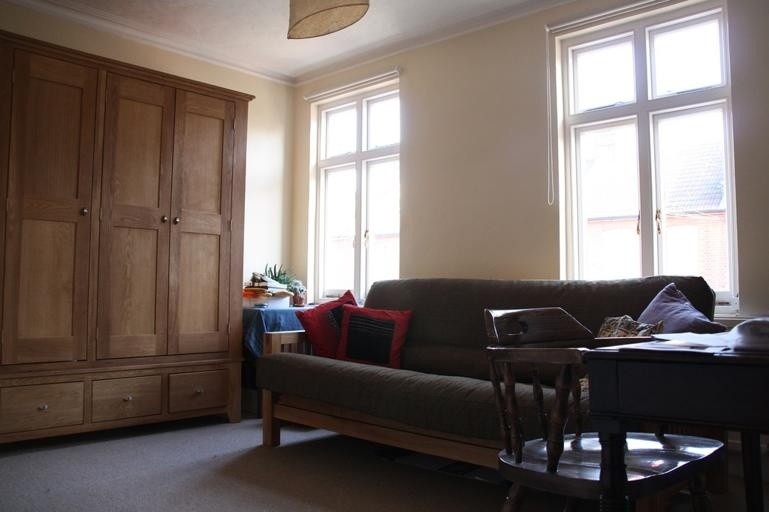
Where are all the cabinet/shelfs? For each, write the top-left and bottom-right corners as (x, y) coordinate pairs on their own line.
(0, 29), (255, 450)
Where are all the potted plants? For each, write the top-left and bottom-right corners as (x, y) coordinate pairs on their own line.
(289, 280), (306, 306)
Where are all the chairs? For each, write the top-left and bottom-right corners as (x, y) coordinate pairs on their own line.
(485, 307), (727, 512)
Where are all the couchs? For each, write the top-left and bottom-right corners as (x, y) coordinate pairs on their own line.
(257, 275), (717, 469)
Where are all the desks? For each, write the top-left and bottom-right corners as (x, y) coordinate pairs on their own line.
(243, 309), (304, 419)
(576, 332), (769, 511)
